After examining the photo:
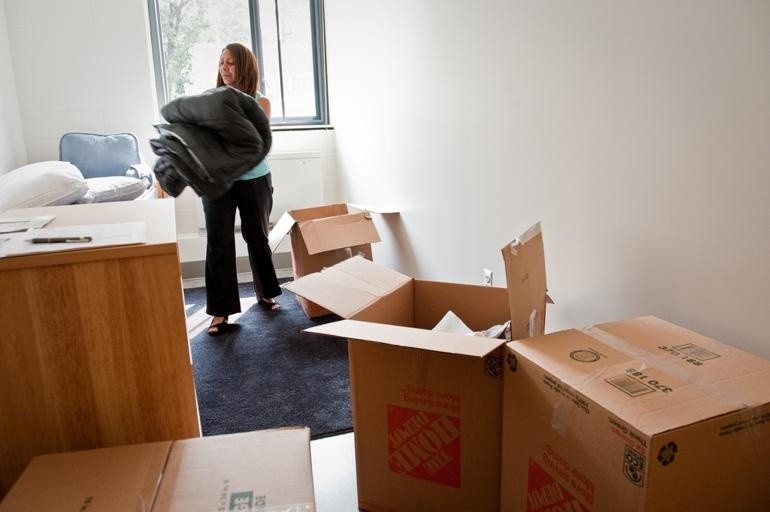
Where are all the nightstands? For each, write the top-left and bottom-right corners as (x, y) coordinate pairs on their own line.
(0, 196), (203, 498)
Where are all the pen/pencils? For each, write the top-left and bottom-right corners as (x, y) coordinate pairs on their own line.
(24, 236), (93, 243)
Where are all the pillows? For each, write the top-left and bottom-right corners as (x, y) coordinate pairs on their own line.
(126, 165), (153, 189)
(76, 176), (150, 204)
(60, 132), (138, 178)
(0, 161), (89, 215)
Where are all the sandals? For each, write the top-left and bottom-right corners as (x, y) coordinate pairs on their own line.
(255, 293), (280, 313)
(207, 312), (228, 338)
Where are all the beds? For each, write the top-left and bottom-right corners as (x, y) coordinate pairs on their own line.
(135, 179), (168, 200)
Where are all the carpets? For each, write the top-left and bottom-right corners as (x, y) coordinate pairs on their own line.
(184, 276), (354, 439)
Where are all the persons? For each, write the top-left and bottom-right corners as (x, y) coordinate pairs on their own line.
(156, 43), (285, 336)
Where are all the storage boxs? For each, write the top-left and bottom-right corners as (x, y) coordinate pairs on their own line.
(500, 315), (770, 512)
(0, 432), (172, 512)
(267, 203), (400, 317)
(152, 426), (315, 512)
(278, 222), (555, 512)
